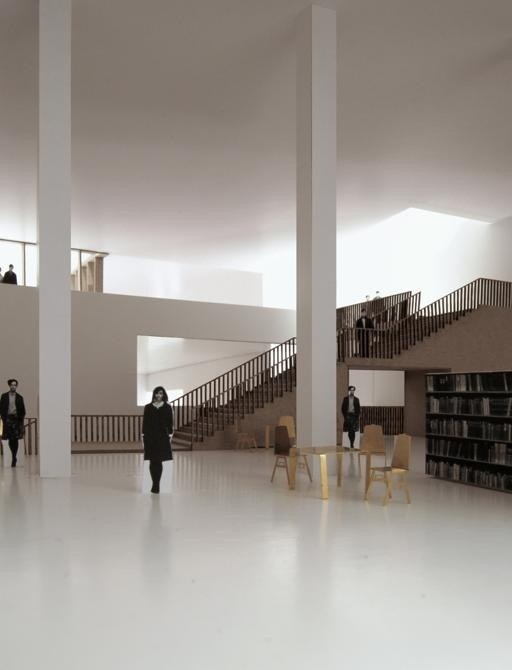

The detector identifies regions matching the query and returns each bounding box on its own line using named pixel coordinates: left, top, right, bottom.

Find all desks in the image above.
left=288, top=446, right=372, bottom=500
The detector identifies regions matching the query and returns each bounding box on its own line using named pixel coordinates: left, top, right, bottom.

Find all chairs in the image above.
left=278, top=416, right=296, bottom=439
left=358, top=424, right=387, bottom=478
left=271, top=426, right=312, bottom=487
left=235, top=415, right=258, bottom=450
left=365, top=433, right=412, bottom=506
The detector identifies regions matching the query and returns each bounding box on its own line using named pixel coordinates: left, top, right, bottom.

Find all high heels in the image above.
left=11, top=459, right=17, bottom=466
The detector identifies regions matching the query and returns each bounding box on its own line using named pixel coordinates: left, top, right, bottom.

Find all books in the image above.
left=426, top=372, right=512, bottom=490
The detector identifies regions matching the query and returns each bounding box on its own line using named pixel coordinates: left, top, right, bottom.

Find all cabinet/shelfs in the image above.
left=424, top=370, right=511, bottom=494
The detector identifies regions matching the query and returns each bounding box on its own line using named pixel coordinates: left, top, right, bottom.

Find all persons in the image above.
left=374, top=291, right=381, bottom=301
left=355, top=308, right=374, bottom=357
left=366, top=295, right=370, bottom=301
left=3, top=264, right=16, bottom=284
left=0, top=379, right=25, bottom=467
left=342, top=386, right=360, bottom=448
left=142, top=387, right=173, bottom=493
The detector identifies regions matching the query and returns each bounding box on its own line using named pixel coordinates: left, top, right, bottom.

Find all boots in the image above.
left=151, top=474, right=161, bottom=493
